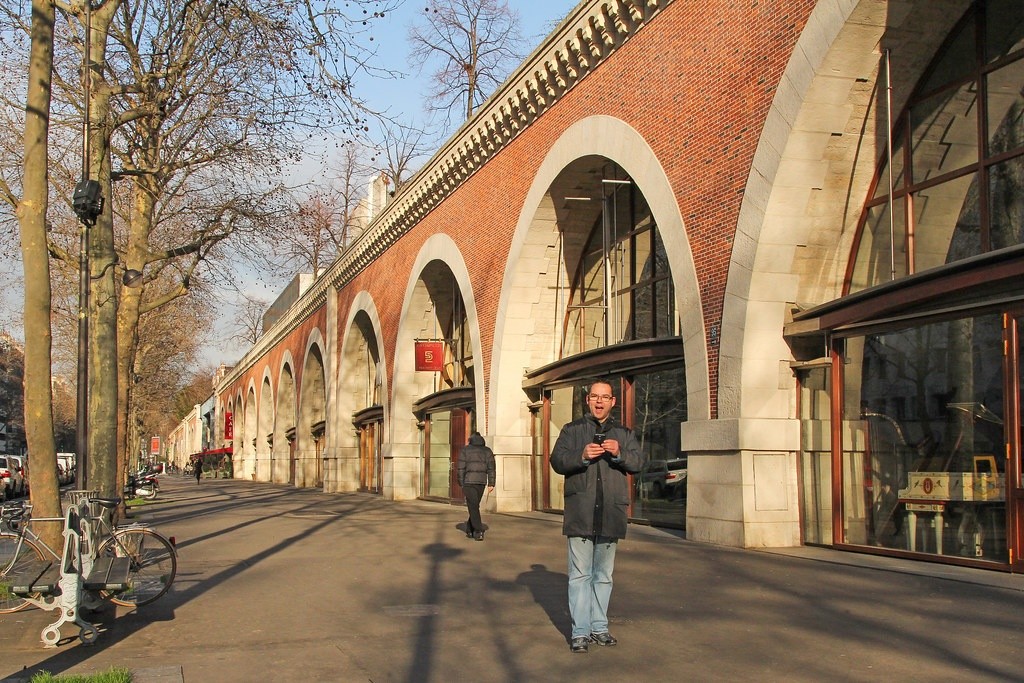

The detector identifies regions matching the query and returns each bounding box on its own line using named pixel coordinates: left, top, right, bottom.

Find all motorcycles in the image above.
left=124, top=464, right=163, bottom=500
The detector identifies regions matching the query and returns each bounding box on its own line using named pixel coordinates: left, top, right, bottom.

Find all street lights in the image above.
left=73, top=1, right=145, bottom=518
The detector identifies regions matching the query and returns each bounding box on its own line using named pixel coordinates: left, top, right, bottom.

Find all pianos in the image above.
left=897, top=471, right=1024, bottom=555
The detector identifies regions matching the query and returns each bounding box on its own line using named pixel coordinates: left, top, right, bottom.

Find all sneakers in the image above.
left=571, top=636, right=589, bottom=653
left=589, top=630, right=617, bottom=645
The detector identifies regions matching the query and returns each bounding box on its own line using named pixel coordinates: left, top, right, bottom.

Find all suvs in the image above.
left=634, top=457, right=689, bottom=496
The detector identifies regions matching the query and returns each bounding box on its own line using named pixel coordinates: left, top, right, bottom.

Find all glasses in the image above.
left=588, top=394, right=613, bottom=402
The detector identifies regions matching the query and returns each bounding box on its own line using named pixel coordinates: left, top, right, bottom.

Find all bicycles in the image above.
left=0, top=498, right=180, bottom=614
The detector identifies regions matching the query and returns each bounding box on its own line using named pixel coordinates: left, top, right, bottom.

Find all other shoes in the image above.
left=466, top=532, right=474, bottom=538
left=476, top=533, right=483, bottom=541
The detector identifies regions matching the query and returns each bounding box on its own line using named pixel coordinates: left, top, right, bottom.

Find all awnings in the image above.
left=199, top=446, right=234, bottom=457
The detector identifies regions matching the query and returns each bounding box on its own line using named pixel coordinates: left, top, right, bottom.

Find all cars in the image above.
left=56, top=453, right=77, bottom=485
left=0, top=451, right=30, bottom=501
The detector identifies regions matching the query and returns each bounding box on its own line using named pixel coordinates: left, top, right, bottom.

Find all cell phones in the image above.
left=593, top=434, right=606, bottom=447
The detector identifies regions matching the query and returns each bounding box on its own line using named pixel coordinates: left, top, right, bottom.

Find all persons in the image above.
left=548, top=377, right=644, bottom=654
left=457, top=432, right=496, bottom=542
left=195, top=459, right=202, bottom=485
